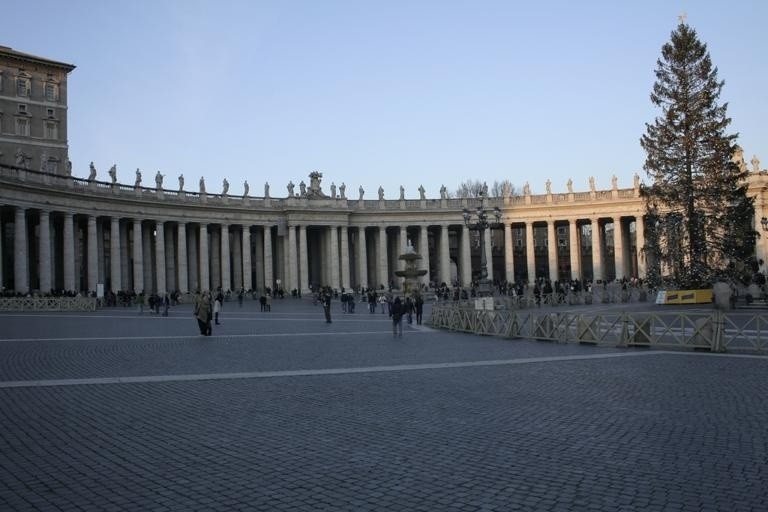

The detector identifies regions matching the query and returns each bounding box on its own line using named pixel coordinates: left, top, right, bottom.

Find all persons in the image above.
left=329, top=168, right=664, bottom=200
left=0, top=287, right=181, bottom=318
left=429, top=272, right=657, bottom=306
left=14, top=150, right=307, bottom=199
left=309, top=280, right=427, bottom=336
left=227, top=284, right=300, bottom=312
left=192, top=285, right=225, bottom=336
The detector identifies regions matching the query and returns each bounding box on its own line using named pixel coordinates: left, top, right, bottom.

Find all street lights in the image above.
left=460, top=183, right=504, bottom=322
left=760, top=215, right=768, bottom=240
